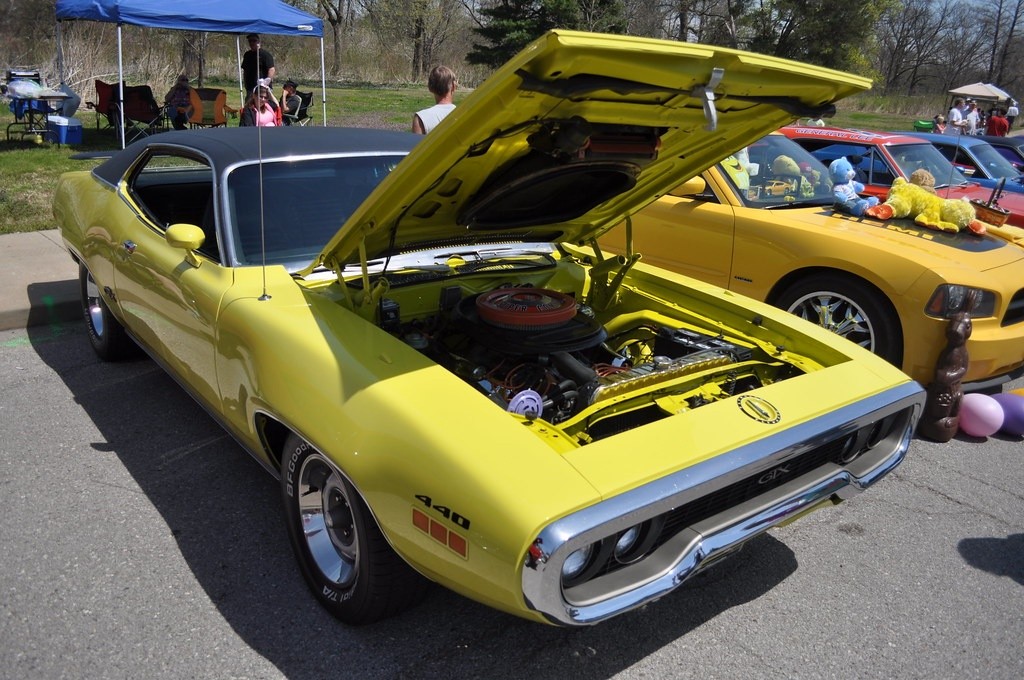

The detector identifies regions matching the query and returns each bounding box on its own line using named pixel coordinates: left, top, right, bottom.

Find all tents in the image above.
left=55, top=0, right=327, bottom=151
left=943, top=81, right=1018, bottom=116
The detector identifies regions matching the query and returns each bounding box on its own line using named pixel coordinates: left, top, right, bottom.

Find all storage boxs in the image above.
left=44, top=115, right=83, bottom=145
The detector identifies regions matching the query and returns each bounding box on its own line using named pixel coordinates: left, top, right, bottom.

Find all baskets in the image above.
left=968, top=177, right=1012, bottom=228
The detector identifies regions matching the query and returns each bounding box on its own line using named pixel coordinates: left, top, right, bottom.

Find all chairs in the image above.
left=278, top=190, right=348, bottom=248
left=847, top=153, right=868, bottom=184
left=5, top=71, right=314, bottom=144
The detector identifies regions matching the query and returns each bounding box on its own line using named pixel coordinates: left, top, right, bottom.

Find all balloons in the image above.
left=958, top=393, right=1004, bottom=437
left=989, top=393, right=1024, bottom=437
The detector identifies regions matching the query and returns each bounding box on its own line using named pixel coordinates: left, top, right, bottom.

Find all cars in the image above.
left=774, top=125, right=1024, bottom=230
left=575, top=129, right=1024, bottom=404
left=807, top=130, right=1024, bottom=196
left=960, top=133, right=1024, bottom=176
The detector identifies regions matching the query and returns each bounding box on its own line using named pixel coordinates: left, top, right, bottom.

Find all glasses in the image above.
left=260, top=95, right=268, bottom=99
left=180, top=78, right=188, bottom=82
left=248, top=39, right=259, bottom=43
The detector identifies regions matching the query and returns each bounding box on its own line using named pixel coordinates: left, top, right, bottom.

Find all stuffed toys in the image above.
left=721, top=156, right=750, bottom=198
left=772, top=155, right=820, bottom=198
left=869, top=169, right=986, bottom=237
left=828, top=156, right=879, bottom=216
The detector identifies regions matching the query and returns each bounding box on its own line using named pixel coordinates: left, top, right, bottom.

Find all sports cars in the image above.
left=49, top=26, right=930, bottom=631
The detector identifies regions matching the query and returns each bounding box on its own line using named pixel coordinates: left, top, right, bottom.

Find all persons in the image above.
left=280, top=81, right=299, bottom=128
left=240, top=32, right=275, bottom=102
left=807, top=116, right=825, bottom=127
left=164, top=75, right=200, bottom=130
left=412, top=65, right=457, bottom=135
left=935, top=97, right=1019, bottom=136
left=240, top=79, right=279, bottom=127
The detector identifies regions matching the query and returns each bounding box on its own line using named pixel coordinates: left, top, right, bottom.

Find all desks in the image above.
left=7, top=80, right=73, bottom=148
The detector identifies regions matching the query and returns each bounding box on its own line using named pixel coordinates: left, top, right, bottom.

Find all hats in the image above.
left=287, top=78, right=298, bottom=89
left=246, top=33, right=259, bottom=39
left=968, top=99, right=977, bottom=105
